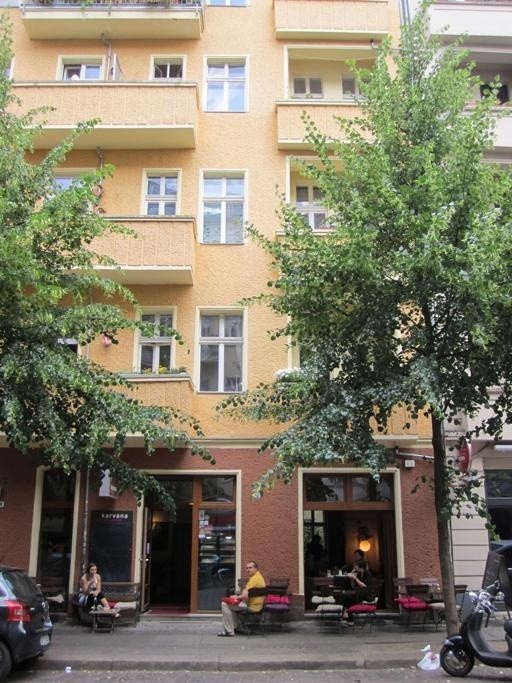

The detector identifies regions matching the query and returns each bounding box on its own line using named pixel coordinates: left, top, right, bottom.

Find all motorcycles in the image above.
left=440, top=579, right=512, bottom=677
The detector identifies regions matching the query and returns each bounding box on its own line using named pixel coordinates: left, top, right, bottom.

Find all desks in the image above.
left=89, top=609, right=120, bottom=634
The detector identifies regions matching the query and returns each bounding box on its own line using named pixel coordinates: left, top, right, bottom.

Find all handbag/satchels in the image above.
left=71, top=591, right=89, bottom=606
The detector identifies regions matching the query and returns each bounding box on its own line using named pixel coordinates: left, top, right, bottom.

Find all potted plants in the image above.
left=179, top=367, right=187, bottom=374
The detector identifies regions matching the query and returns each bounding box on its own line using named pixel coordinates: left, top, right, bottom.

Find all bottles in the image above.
left=327, top=569, right=348, bottom=577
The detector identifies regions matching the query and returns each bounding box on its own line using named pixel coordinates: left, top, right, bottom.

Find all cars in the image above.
left=0, top=563, right=55, bottom=682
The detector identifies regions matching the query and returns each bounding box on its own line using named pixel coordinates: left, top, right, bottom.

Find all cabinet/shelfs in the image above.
left=195, top=525, right=236, bottom=568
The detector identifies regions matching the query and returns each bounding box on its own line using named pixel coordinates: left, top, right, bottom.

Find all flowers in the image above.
left=273, top=367, right=315, bottom=382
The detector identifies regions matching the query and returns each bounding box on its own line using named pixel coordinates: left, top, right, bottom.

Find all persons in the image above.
left=216, top=558, right=267, bottom=637
left=343, top=547, right=370, bottom=604
left=339, top=559, right=380, bottom=627
left=305, top=533, right=328, bottom=591
left=76, top=560, right=122, bottom=624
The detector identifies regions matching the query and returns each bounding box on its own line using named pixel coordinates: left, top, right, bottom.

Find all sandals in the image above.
left=217, top=630, right=234, bottom=635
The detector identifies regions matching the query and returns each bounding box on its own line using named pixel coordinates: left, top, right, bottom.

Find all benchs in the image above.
left=72, top=581, right=141, bottom=628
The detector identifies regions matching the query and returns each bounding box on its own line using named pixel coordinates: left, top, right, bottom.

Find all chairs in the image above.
left=26, top=576, right=65, bottom=621
left=237, top=574, right=468, bottom=635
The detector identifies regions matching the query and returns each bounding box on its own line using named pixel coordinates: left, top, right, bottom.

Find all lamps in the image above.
left=357, top=531, right=371, bottom=552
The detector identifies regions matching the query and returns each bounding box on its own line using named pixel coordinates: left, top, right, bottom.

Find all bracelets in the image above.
left=354, top=577, right=357, bottom=581
